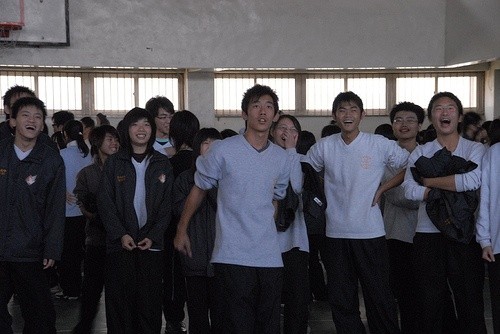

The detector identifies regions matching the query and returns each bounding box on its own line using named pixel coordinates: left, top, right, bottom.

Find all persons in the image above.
left=476, top=142, right=500, bottom=334
left=172, top=128, right=221, bottom=334
left=220, top=129, right=239, bottom=140
left=169, top=111, right=200, bottom=179
left=0, top=97, right=66, bottom=334
left=51, top=130, right=68, bottom=150
left=302, top=91, right=411, bottom=334
left=376, top=124, right=398, bottom=144
left=403, top=92, right=488, bottom=334
left=51, top=111, right=74, bottom=133
left=78, top=117, right=94, bottom=149
left=145, top=96, right=187, bottom=334
left=180, top=84, right=284, bottom=334
left=73, top=125, right=122, bottom=334
left=296, top=129, right=316, bottom=154
left=268, top=115, right=311, bottom=334
left=416, top=111, right=497, bottom=150
left=56, top=121, right=94, bottom=302
left=381, top=103, right=426, bottom=334
left=116, top=120, right=130, bottom=148
left=0, top=86, right=48, bottom=142
left=98, top=107, right=175, bottom=334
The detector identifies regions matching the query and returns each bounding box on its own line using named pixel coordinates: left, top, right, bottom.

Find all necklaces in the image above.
left=310, top=125, right=345, bottom=309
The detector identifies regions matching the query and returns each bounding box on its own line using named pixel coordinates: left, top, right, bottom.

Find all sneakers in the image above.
left=50, top=286, right=80, bottom=301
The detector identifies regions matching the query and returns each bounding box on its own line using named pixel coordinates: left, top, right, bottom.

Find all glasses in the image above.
left=275, top=125, right=298, bottom=133
left=157, top=115, right=175, bottom=119
left=52, top=123, right=57, bottom=127
left=432, top=105, right=459, bottom=112
left=131, top=123, right=151, bottom=128
left=394, top=118, right=418, bottom=124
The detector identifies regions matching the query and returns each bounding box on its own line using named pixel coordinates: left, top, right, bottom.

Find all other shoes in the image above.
left=165, top=321, right=188, bottom=334
